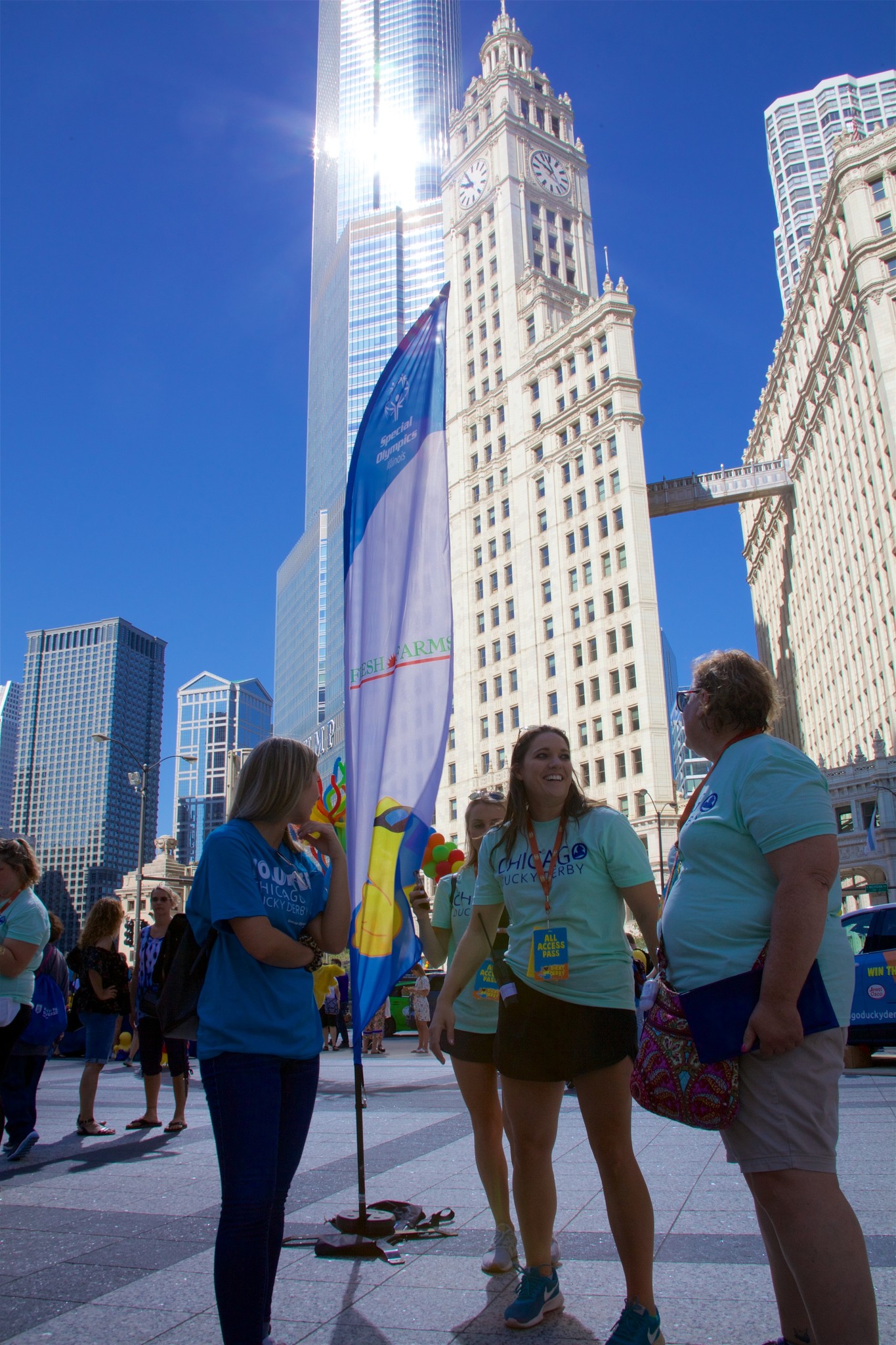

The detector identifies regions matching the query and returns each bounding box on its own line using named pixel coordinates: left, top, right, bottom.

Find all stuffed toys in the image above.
left=312, top=964, right=345, bottom=1010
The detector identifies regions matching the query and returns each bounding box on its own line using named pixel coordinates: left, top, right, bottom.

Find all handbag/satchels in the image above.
left=409, top=996, right=418, bottom=1030
left=15, top=944, right=68, bottom=1057
left=628, top=979, right=741, bottom=1131
left=676, top=959, right=840, bottom=1063
left=325, top=996, right=340, bottom=1015
left=136, top=912, right=217, bottom=1029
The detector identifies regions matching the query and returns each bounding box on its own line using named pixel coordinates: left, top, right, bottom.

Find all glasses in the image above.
left=517, top=725, right=566, bottom=742
left=676, top=688, right=702, bottom=710
left=275, top=850, right=310, bottom=889
left=151, top=897, right=172, bottom=902
left=468, top=791, right=507, bottom=807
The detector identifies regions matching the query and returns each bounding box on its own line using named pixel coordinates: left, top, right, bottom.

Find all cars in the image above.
left=383, top=969, right=446, bottom=1034
left=837, top=903, right=896, bottom=1048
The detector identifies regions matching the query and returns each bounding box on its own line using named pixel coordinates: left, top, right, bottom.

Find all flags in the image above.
left=864, top=804, right=877, bottom=856
left=341, top=305, right=453, bottom=1028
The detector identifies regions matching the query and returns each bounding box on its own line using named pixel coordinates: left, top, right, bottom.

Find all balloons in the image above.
left=291, top=757, right=346, bottom=890
left=420, top=826, right=466, bottom=883
left=424, top=961, right=429, bottom=969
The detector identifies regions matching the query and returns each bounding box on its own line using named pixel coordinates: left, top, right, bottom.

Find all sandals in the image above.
left=332, top=1046, right=339, bottom=1051
left=322, top=1046, right=329, bottom=1051
left=76, top=1114, right=107, bottom=1126
left=77, top=1118, right=116, bottom=1136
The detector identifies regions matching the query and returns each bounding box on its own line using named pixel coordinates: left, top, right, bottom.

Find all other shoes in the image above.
left=328, top=1039, right=336, bottom=1046
left=367, top=1041, right=373, bottom=1050
left=378, top=1044, right=385, bottom=1052
left=371, top=1049, right=383, bottom=1054
left=361, top=1049, right=368, bottom=1053
left=337, top=1043, right=349, bottom=1048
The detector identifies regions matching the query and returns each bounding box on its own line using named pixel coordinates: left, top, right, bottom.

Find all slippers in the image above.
left=126, top=1118, right=162, bottom=1130
left=164, top=1120, right=188, bottom=1133
left=416, top=1048, right=428, bottom=1053
left=410, top=1048, right=419, bottom=1053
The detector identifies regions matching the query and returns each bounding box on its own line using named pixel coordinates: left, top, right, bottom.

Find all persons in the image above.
left=186, top=738, right=350, bottom=1345
left=65, top=896, right=130, bottom=1136
left=3, top=911, right=69, bottom=1151
left=429, top=725, right=663, bottom=1345
left=318, top=958, right=350, bottom=1052
left=50, top=965, right=198, bottom=1062
left=126, top=886, right=192, bottom=1133
left=361, top=995, right=391, bottom=1054
left=0, top=837, right=51, bottom=1161
left=409, top=786, right=560, bottom=1275
left=657, top=646, right=880, bottom=1345
left=624, top=932, right=657, bottom=1049
left=402, top=963, right=430, bottom=1054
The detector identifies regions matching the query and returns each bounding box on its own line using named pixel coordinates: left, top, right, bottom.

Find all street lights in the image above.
left=639, top=788, right=679, bottom=898
left=91, top=733, right=198, bottom=1020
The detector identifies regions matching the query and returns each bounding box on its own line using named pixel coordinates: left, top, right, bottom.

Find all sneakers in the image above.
left=3, top=1141, right=14, bottom=1151
left=123, top=1057, right=133, bottom=1066
left=603, top=1298, right=666, bottom=1345
left=480, top=1223, right=518, bottom=1272
left=550, top=1233, right=561, bottom=1264
left=6, top=1128, right=40, bottom=1161
left=503, top=1263, right=564, bottom=1329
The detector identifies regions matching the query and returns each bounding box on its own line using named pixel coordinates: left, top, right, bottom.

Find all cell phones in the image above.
left=413, top=869, right=430, bottom=910
